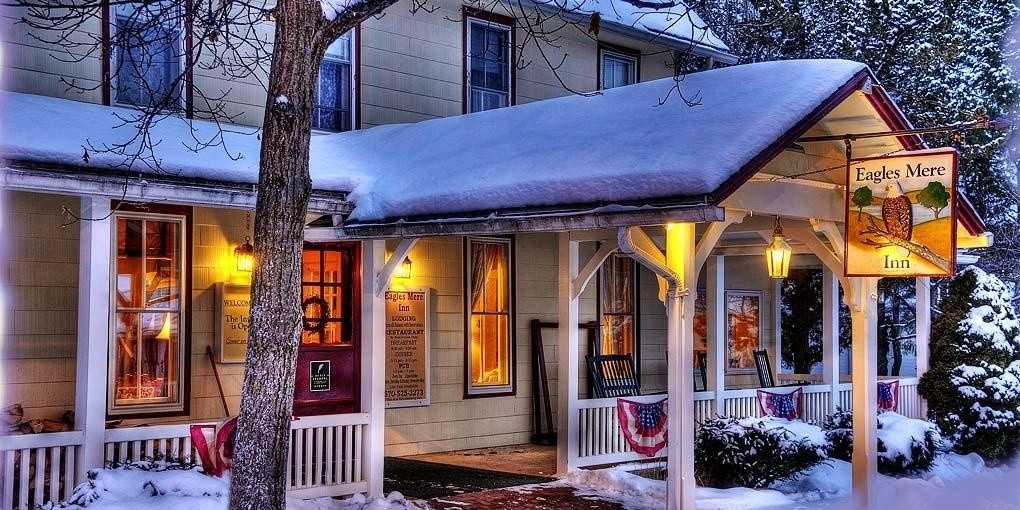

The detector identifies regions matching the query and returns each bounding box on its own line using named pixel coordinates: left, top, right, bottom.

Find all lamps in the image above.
left=394, top=237, right=411, bottom=280
left=764, top=216, right=792, bottom=279
left=234, top=211, right=255, bottom=272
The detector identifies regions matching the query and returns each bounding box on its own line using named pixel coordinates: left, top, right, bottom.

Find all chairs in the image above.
left=665, top=351, right=704, bottom=391
left=752, top=348, right=811, bottom=386
left=585, top=353, right=667, bottom=397
left=698, top=351, right=741, bottom=390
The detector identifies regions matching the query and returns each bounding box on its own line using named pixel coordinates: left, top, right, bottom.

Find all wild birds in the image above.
left=880, top=179, right=914, bottom=243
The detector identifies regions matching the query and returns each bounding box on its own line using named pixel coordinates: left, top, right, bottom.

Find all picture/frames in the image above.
left=214, top=280, right=251, bottom=364
left=693, top=288, right=708, bottom=374
left=723, top=288, right=764, bottom=376
left=386, top=284, right=431, bottom=409
